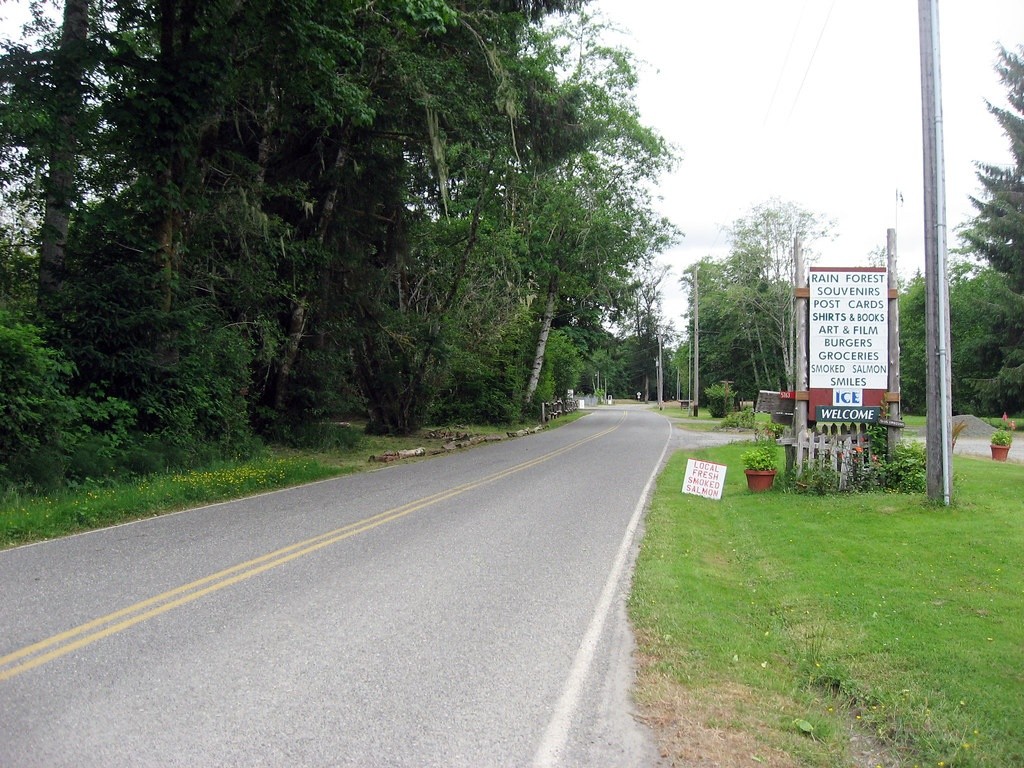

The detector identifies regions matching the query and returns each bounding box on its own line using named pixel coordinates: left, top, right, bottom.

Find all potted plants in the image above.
left=742, top=446, right=777, bottom=490
left=990, top=428, right=1012, bottom=462
left=952, top=421, right=968, bottom=452
